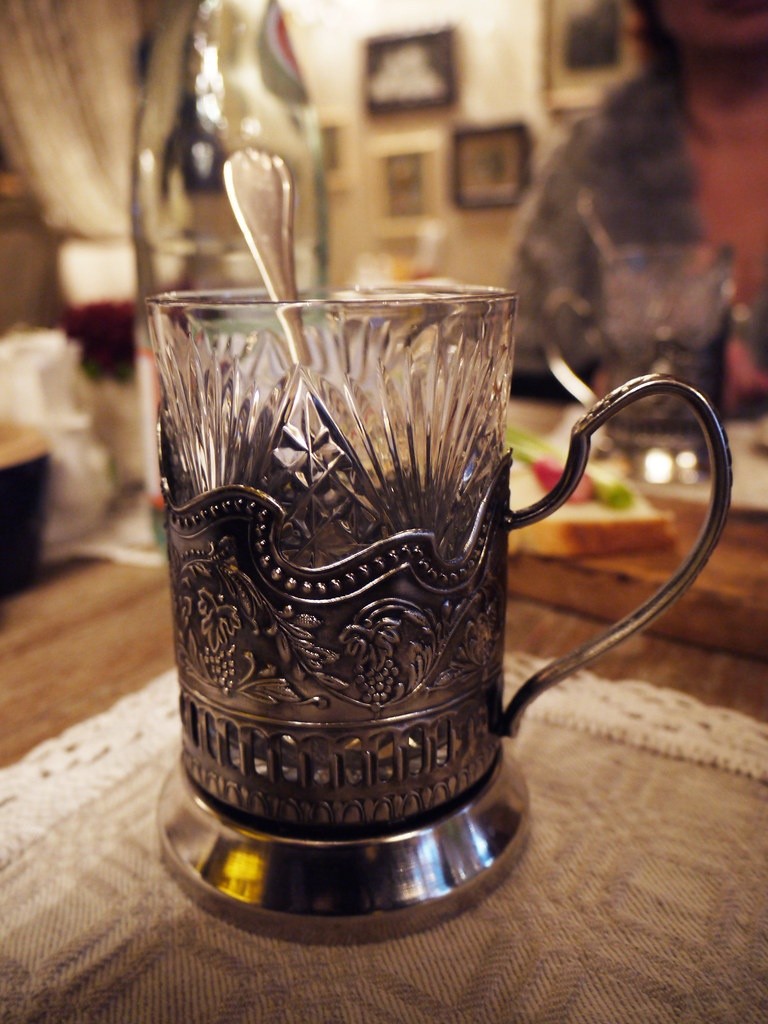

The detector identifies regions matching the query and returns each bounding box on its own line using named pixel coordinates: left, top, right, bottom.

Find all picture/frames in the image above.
left=374, top=151, right=434, bottom=215
left=454, top=124, right=532, bottom=211
left=540, top=0, right=648, bottom=118
left=364, top=29, right=456, bottom=117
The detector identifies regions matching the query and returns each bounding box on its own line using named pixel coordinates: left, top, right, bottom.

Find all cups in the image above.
left=536, top=238, right=738, bottom=488
left=142, top=284, right=734, bottom=943
